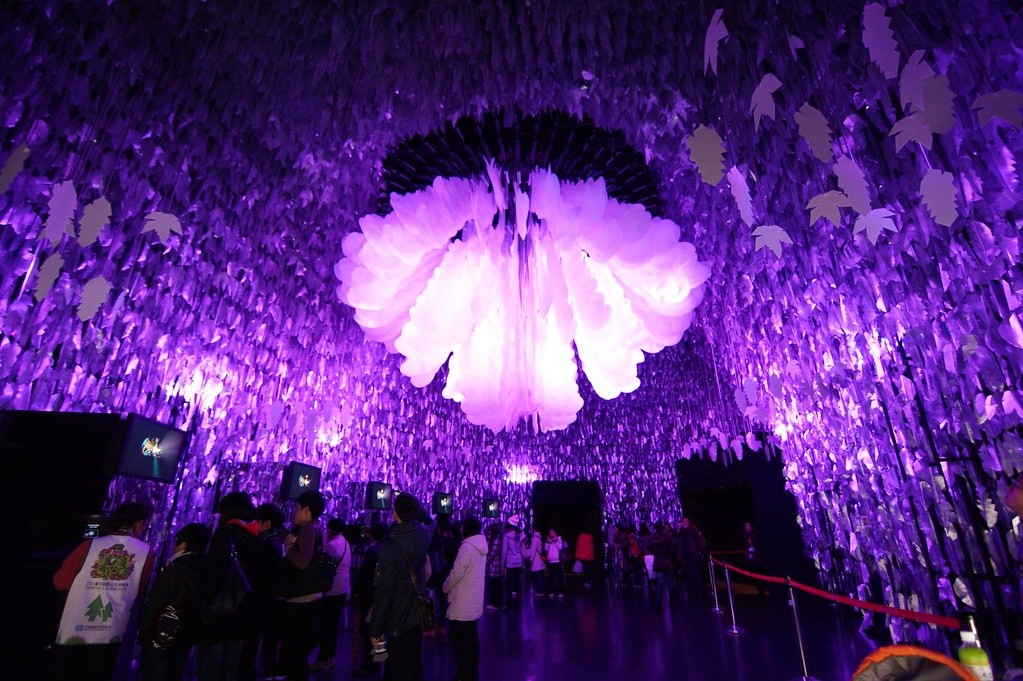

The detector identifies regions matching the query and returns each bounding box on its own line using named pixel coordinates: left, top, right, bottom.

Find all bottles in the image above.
left=959, top=631, right=993, bottom=681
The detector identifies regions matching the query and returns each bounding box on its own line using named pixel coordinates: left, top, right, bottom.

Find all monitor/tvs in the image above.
left=285, top=461, right=322, bottom=501
left=116, top=412, right=187, bottom=484
left=483, top=499, right=500, bottom=518
left=364, top=481, right=393, bottom=510
left=432, top=493, right=454, bottom=514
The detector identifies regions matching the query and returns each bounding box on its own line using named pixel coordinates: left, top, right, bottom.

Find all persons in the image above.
left=51, top=491, right=708, bottom=681
left=738, top=521, right=769, bottom=597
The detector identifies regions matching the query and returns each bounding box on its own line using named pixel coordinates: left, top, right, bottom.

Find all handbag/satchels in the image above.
left=419, top=586, right=440, bottom=631
left=282, top=520, right=336, bottom=599
left=156, top=596, right=195, bottom=647
left=207, top=530, right=251, bottom=619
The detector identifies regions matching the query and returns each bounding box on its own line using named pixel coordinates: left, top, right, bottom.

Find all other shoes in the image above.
left=352, top=667, right=369, bottom=677
left=308, top=659, right=331, bottom=671
left=487, top=605, right=499, bottom=610
left=329, top=657, right=337, bottom=666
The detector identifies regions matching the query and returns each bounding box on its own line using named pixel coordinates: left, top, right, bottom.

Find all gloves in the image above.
left=371, top=639, right=389, bottom=662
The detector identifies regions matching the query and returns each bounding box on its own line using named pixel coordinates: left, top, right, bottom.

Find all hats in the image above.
left=328, top=518, right=345, bottom=533
left=507, top=513, right=520, bottom=527
left=395, top=493, right=420, bottom=522
